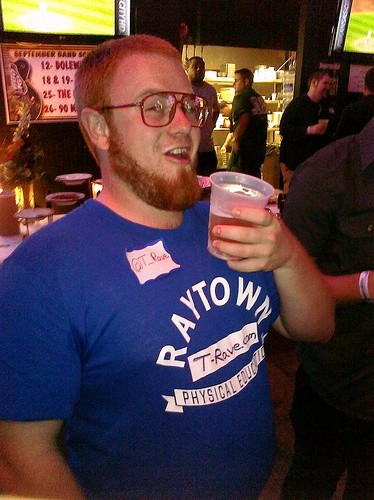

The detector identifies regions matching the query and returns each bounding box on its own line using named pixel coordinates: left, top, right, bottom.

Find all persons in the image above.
left=0, top=36, right=337, bottom=500
left=184, top=57, right=374, bottom=221
left=282, top=117, right=374, bottom=500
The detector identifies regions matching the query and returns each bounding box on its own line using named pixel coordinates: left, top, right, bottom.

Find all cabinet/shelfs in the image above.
left=203, top=53, right=296, bottom=189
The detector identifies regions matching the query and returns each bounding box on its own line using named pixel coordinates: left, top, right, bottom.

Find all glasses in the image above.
left=94, top=91, right=210, bottom=128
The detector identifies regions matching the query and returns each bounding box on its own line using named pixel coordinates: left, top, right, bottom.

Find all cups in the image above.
left=206, top=171, right=274, bottom=262
left=318, top=119, right=329, bottom=133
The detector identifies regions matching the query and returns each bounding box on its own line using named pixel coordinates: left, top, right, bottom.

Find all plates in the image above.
left=55, top=173, right=93, bottom=186
left=14, top=208, right=55, bottom=221
left=45, top=192, right=85, bottom=205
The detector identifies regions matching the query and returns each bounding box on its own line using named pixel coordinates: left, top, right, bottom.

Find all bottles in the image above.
left=267, top=111, right=272, bottom=128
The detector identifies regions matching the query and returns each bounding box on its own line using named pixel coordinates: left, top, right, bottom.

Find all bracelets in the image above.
left=307, top=125, right=312, bottom=135
left=359, top=271, right=371, bottom=303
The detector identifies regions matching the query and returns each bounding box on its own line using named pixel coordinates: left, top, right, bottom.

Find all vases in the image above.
left=13, top=179, right=47, bottom=210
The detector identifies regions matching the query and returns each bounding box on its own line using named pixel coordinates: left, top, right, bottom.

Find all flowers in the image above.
left=0, top=96, right=46, bottom=191
